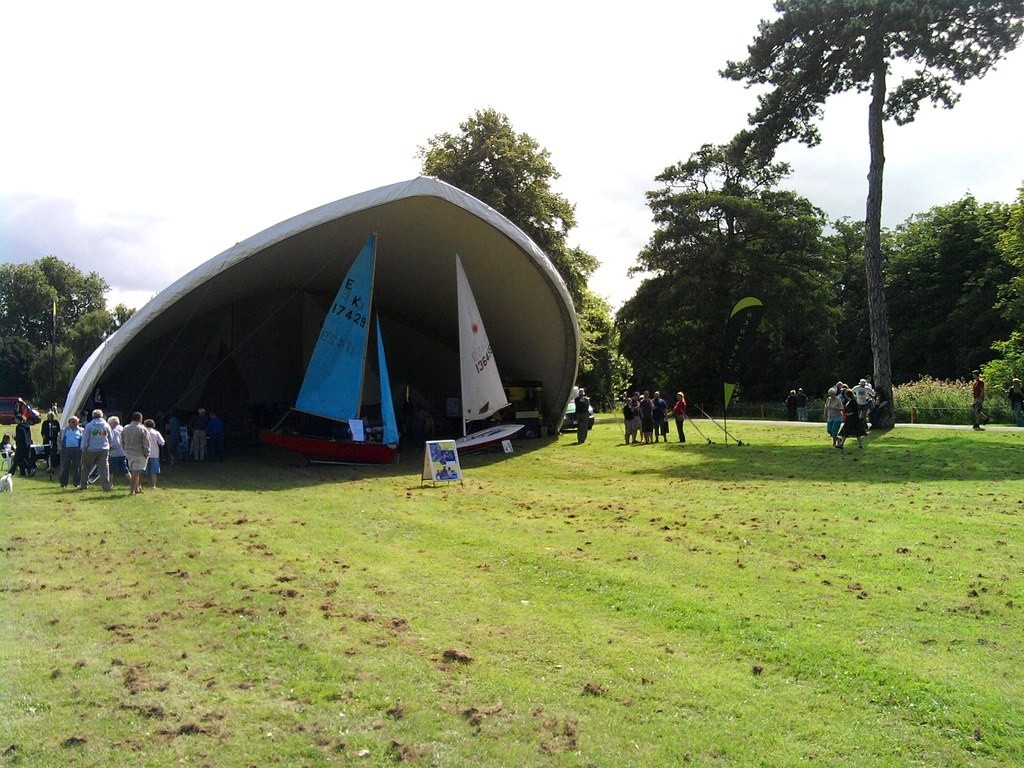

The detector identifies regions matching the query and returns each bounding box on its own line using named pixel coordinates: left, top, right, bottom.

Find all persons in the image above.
left=1006, top=379, right=1024, bottom=427
left=667, top=392, right=686, bottom=443
left=205, top=411, right=224, bottom=462
left=145, top=419, right=165, bottom=490
left=191, top=408, right=207, bottom=461
left=823, top=375, right=888, bottom=449
left=0, top=414, right=38, bottom=478
left=575, top=389, right=589, bottom=444
left=158, top=412, right=180, bottom=464
left=786, top=388, right=808, bottom=422
left=403, top=396, right=434, bottom=449
left=119, top=412, right=150, bottom=496
left=624, top=391, right=667, bottom=445
left=972, top=370, right=984, bottom=429
left=77, top=409, right=113, bottom=492
left=77, top=411, right=100, bottom=485
left=41, top=412, right=60, bottom=474
left=53, top=403, right=58, bottom=414
left=107, top=416, right=132, bottom=489
left=60, top=416, right=85, bottom=487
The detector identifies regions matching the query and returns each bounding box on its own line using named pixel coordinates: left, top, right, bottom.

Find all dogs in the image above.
left=0, top=474, right=13, bottom=492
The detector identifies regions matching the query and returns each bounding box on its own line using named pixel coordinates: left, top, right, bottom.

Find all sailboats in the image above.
left=451, top=251, right=528, bottom=457
left=255, top=229, right=403, bottom=464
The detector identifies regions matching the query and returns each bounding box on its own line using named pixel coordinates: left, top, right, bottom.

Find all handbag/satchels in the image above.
left=677, top=415, right=685, bottom=420
left=660, top=420, right=669, bottom=435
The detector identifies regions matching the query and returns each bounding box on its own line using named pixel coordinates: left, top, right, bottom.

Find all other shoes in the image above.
left=983, top=418, right=990, bottom=425
left=136, top=489, right=144, bottom=494
left=973, top=424, right=980, bottom=428
left=835, top=445, right=843, bottom=450
left=128, top=493, right=138, bottom=498
left=859, top=445, right=864, bottom=449
left=650, top=441, right=653, bottom=444
left=76, top=486, right=81, bottom=493
left=645, top=441, right=650, bottom=444
left=60, top=486, right=67, bottom=492
left=30, top=468, right=36, bottom=477
left=110, top=485, right=114, bottom=490
left=16, top=474, right=26, bottom=478
left=641, top=441, right=644, bottom=444
left=149, top=485, right=156, bottom=491
left=632, top=439, right=640, bottom=444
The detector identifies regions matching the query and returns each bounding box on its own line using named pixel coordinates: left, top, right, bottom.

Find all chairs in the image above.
left=0, top=452, right=13, bottom=471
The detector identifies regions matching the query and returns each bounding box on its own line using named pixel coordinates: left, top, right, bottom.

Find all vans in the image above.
left=0, top=396, right=42, bottom=425
left=560, top=385, right=596, bottom=431
